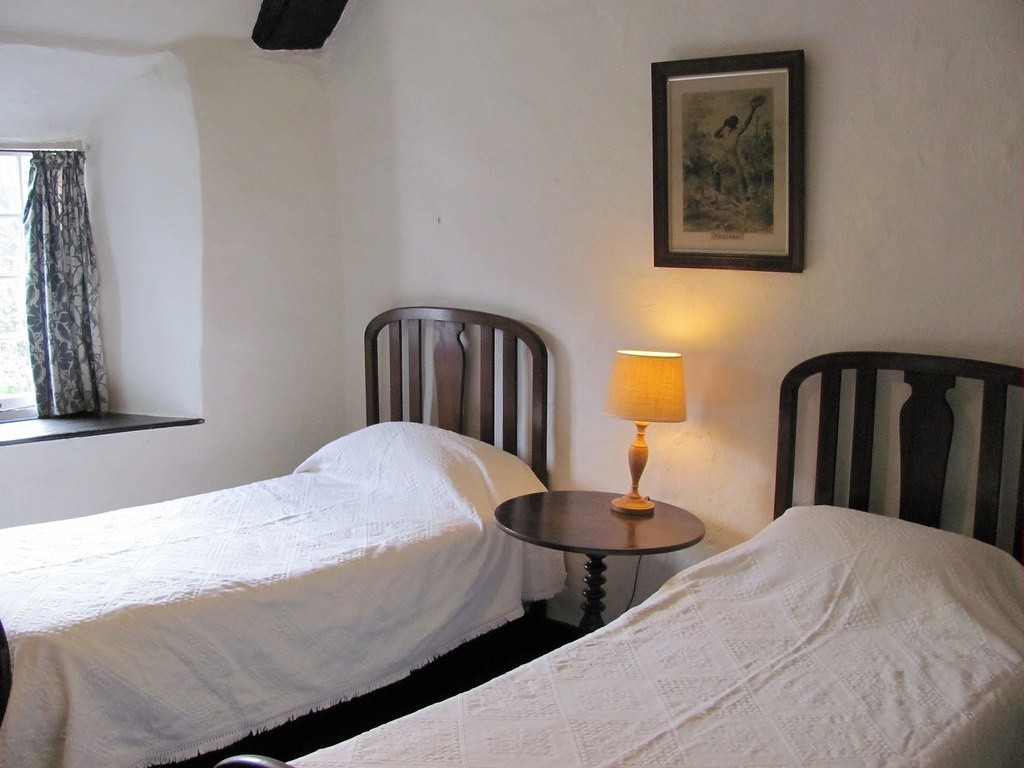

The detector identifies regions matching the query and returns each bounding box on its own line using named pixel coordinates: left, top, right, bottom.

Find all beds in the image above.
left=288, top=505, right=1024, bottom=768
left=1, top=420, right=536, bottom=766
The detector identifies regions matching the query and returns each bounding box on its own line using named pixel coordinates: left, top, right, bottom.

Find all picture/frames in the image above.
left=650, top=47, right=806, bottom=274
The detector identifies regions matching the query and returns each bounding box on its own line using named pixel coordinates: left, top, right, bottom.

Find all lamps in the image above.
left=604, top=350, right=687, bottom=517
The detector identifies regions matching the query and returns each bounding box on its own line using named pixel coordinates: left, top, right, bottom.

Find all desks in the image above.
left=495, top=490, right=706, bottom=636
